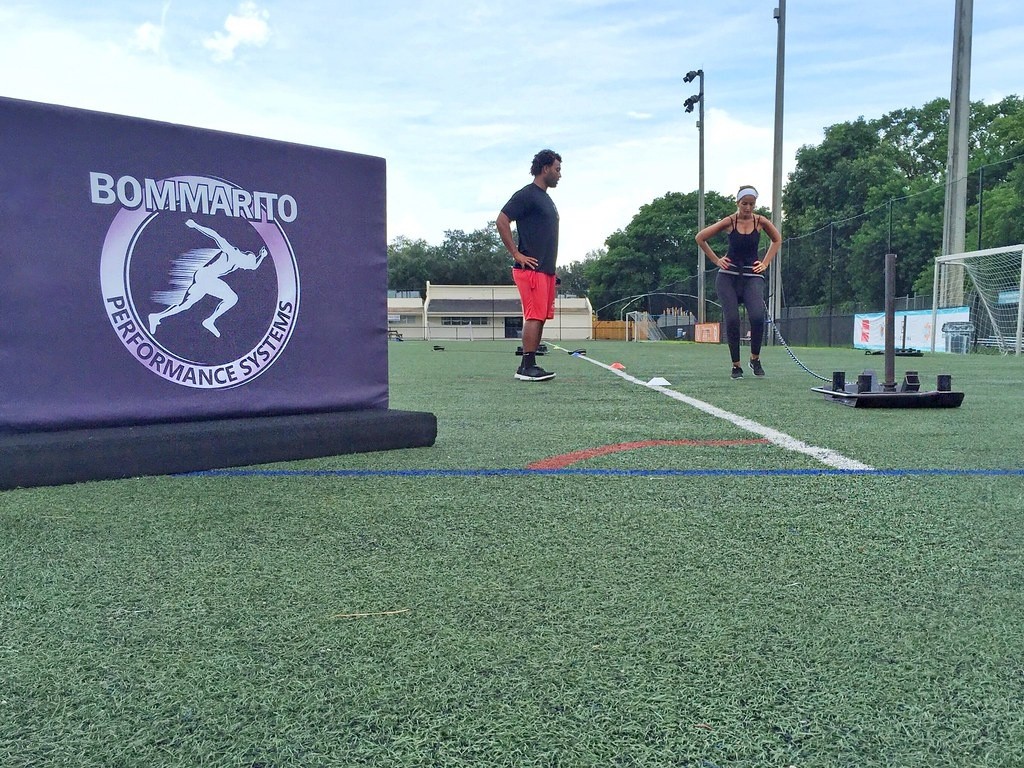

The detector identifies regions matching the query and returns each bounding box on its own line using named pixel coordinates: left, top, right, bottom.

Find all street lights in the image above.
left=682, top=68, right=704, bottom=323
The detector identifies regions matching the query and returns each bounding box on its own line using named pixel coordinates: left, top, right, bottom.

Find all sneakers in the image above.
left=519, top=364, right=556, bottom=381
left=514, top=366, right=523, bottom=378
left=748, top=357, right=766, bottom=377
left=730, top=366, right=744, bottom=380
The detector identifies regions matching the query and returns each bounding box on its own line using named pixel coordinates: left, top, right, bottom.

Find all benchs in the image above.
left=388, top=333, right=403, bottom=339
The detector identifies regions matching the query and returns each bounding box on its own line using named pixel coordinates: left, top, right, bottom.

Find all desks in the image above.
left=387, top=331, right=398, bottom=337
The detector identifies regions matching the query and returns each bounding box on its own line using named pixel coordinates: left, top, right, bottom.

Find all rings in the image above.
left=760, top=267, right=762, bottom=270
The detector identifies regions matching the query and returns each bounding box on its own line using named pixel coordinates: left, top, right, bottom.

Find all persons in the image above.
left=696, top=185, right=781, bottom=378
left=496, top=149, right=563, bottom=380
